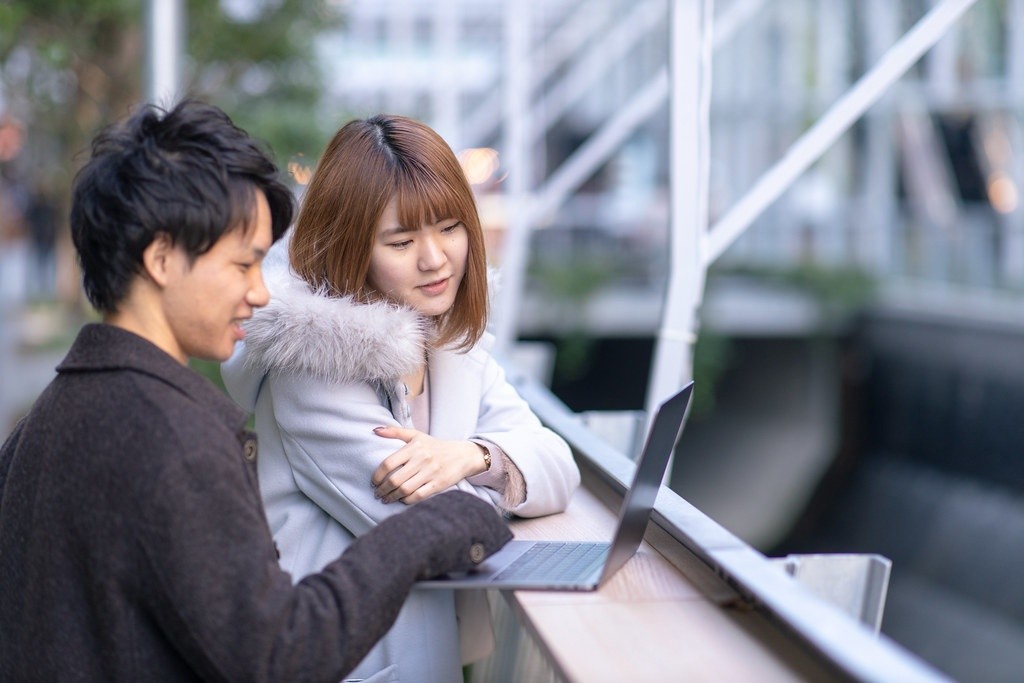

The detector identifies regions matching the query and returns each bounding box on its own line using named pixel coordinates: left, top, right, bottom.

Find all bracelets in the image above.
left=484, top=448, right=491, bottom=470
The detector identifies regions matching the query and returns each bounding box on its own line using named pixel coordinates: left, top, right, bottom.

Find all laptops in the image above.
left=410, top=379, right=696, bottom=592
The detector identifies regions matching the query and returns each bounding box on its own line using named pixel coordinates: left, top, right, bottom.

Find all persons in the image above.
left=1, top=93, right=514, bottom=683
left=219, top=115, right=580, bottom=683
